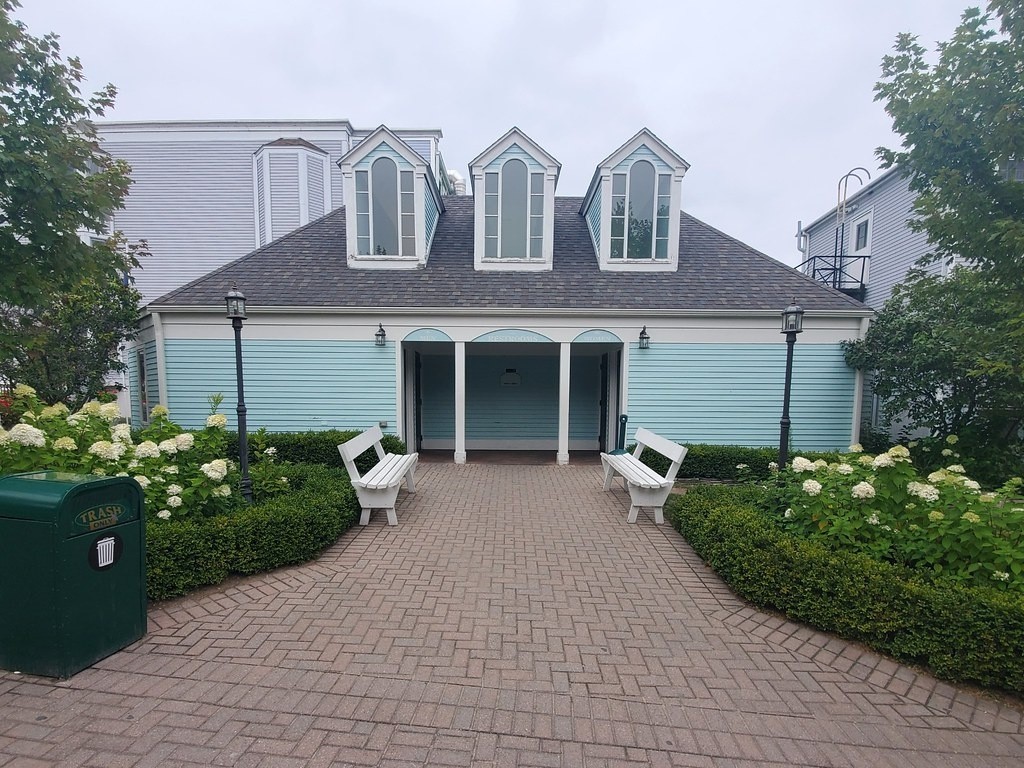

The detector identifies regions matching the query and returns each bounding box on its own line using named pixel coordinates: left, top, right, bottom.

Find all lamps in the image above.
left=639, top=325, right=651, bottom=349
left=375, top=322, right=386, bottom=345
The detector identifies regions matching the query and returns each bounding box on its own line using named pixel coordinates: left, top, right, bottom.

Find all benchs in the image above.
left=337, top=425, right=419, bottom=526
left=600, top=428, right=688, bottom=525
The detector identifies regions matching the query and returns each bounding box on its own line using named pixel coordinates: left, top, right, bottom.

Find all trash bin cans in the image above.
left=0, top=471, right=148, bottom=677
